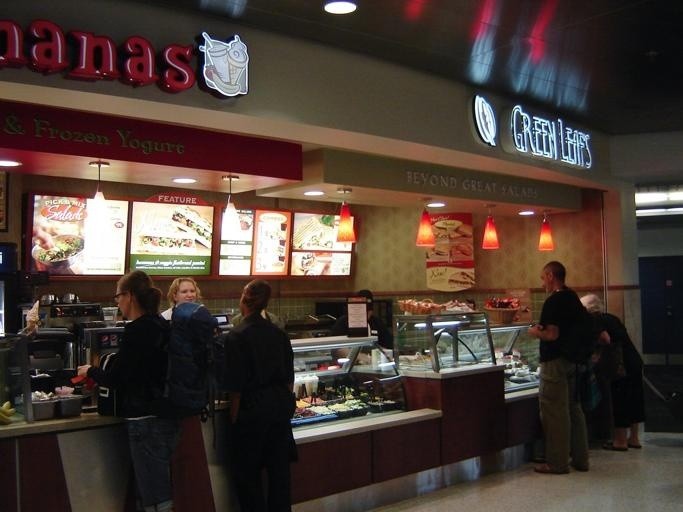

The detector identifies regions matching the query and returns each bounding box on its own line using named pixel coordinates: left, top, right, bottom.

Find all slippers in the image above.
left=603, top=438, right=628, bottom=451
left=625, top=441, right=641, bottom=448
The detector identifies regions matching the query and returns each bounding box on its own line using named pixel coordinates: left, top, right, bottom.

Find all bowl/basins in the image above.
left=32, top=233, right=86, bottom=269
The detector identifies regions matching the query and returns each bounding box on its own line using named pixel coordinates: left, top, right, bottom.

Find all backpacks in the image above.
left=127, top=320, right=211, bottom=419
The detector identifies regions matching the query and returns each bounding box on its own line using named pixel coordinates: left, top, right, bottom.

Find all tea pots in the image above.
left=41, top=292, right=62, bottom=304
left=63, top=292, right=80, bottom=304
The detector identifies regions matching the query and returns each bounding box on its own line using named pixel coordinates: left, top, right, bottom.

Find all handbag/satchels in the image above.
left=98, top=353, right=126, bottom=417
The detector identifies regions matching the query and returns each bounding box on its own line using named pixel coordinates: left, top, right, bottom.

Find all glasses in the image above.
left=112, top=291, right=127, bottom=303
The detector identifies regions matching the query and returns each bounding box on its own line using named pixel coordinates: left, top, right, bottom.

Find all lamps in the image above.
left=336, top=188, right=356, bottom=243
left=220, top=175, right=244, bottom=241
left=482, top=203, right=499, bottom=250
left=85, top=161, right=112, bottom=228
left=415, top=196, right=437, bottom=247
left=538, top=210, right=554, bottom=250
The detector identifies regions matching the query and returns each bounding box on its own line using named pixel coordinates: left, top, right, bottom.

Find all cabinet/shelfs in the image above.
left=170, top=401, right=237, bottom=511
left=504, top=390, right=539, bottom=451
left=392, top=311, right=497, bottom=372
left=457, top=327, right=542, bottom=392
left=407, top=367, right=505, bottom=457
left=0, top=426, right=132, bottom=512
left=292, top=337, right=407, bottom=426
left=289, top=407, right=446, bottom=502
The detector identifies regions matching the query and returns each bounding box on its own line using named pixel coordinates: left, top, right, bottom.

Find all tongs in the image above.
left=300, top=382, right=309, bottom=397
left=310, top=390, right=316, bottom=407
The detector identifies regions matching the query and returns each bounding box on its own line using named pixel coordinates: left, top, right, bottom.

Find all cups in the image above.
left=101, top=306, right=120, bottom=327
left=381, top=350, right=394, bottom=372
left=372, top=349, right=381, bottom=370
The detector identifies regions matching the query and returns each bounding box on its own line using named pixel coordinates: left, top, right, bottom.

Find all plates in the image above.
left=432, top=220, right=465, bottom=230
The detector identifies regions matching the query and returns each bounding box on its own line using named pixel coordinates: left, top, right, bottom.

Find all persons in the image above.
left=0, top=324, right=38, bottom=348
left=580, top=294, right=645, bottom=451
left=331, top=290, right=390, bottom=364
left=94, top=271, right=181, bottom=511
left=223, top=278, right=298, bottom=512
left=229, top=301, right=287, bottom=334
left=527, top=261, right=591, bottom=473
left=161, top=278, right=201, bottom=321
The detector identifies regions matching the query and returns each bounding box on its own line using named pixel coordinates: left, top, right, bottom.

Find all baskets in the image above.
left=398, top=299, right=446, bottom=315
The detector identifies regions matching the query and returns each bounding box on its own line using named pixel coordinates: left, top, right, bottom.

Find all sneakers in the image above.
left=534, top=462, right=553, bottom=474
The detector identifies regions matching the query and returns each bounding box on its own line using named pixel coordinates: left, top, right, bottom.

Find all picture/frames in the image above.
left=0, top=171, right=9, bottom=233
